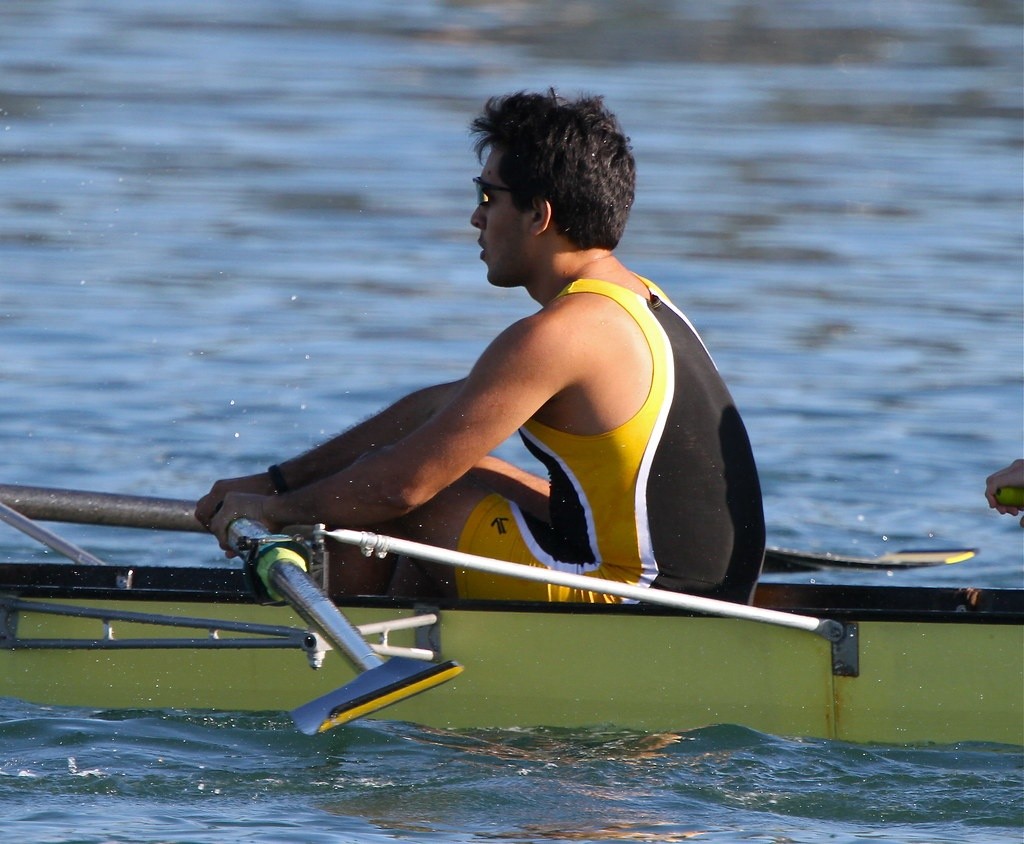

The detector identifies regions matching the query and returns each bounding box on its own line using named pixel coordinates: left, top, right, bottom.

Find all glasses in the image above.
left=472, top=176, right=547, bottom=208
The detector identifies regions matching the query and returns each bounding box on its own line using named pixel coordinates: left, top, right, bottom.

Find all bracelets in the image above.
left=267, top=463, right=290, bottom=497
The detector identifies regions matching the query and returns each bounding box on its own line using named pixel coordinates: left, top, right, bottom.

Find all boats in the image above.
left=0, top=554, right=1023, bottom=751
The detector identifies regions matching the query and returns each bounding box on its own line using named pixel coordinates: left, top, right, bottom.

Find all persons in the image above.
left=982, top=458, right=1023, bottom=517
left=193, top=85, right=767, bottom=620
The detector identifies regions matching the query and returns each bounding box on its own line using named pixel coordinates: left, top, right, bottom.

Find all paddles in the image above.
left=208, top=503, right=466, bottom=738
left=993, top=486, right=1024, bottom=508
left=763, top=545, right=981, bottom=570
